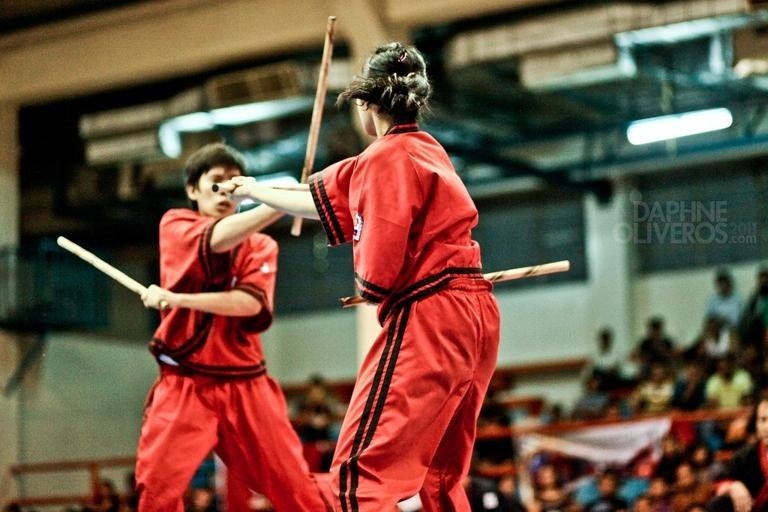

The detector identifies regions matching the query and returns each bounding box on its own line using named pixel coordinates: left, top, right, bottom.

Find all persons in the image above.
left=202, top=37, right=502, bottom=512
left=130, top=140, right=337, bottom=512
